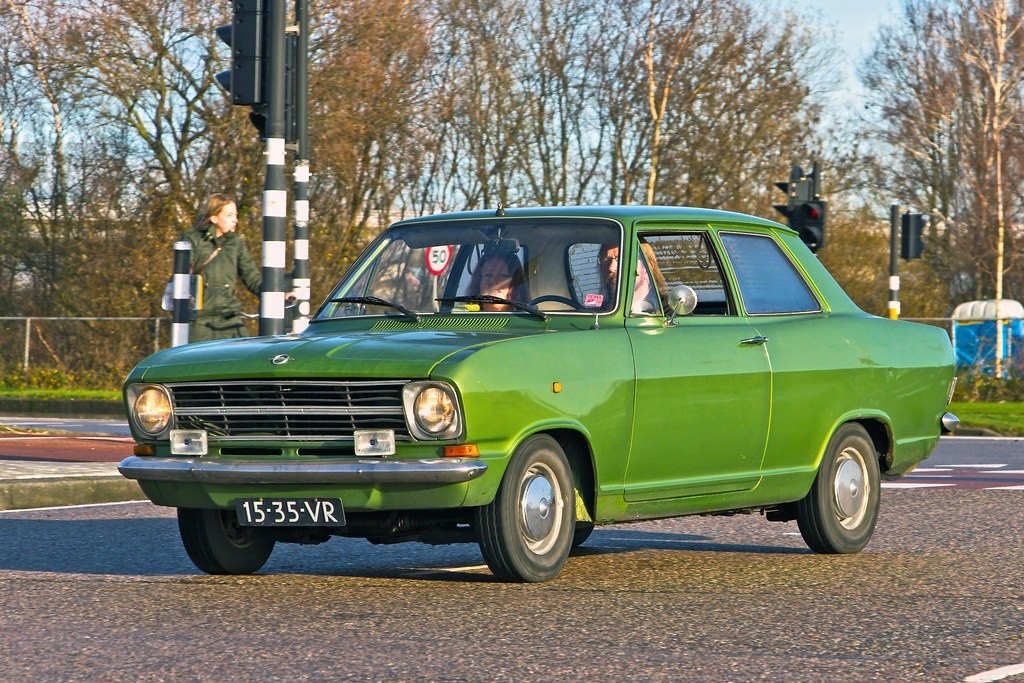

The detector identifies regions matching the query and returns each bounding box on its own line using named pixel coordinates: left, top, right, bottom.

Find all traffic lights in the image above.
left=772, top=167, right=805, bottom=231
left=215, top=0, right=259, bottom=107
left=796, top=199, right=828, bottom=251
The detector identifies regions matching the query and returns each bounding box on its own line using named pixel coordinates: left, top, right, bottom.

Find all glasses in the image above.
left=597, top=256, right=618, bottom=264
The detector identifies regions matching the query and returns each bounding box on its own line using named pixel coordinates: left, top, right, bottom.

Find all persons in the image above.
left=183, top=193, right=261, bottom=343
left=467, top=252, right=531, bottom=313
left=598, top=236, right=694, bottom=317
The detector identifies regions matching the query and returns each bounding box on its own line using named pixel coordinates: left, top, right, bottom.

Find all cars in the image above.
left=114, top=208, right=963, bottom=586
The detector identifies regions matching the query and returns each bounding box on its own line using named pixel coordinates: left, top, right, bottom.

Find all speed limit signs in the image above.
left=426, top=245, right=453, bottom=277
